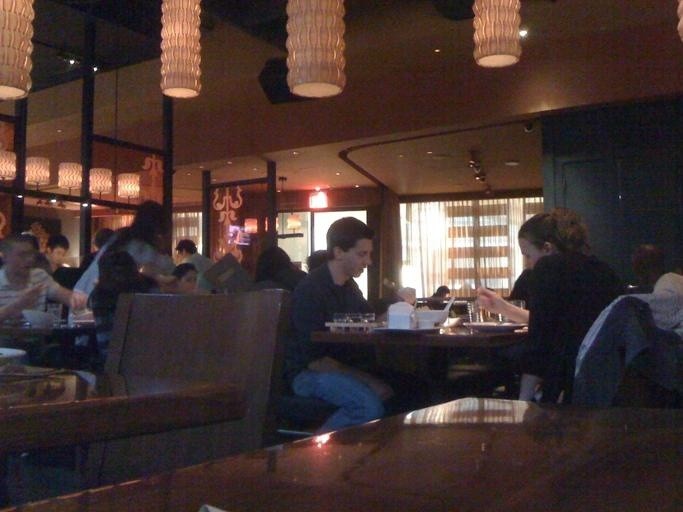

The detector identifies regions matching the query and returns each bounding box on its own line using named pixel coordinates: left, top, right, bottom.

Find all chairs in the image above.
left=102, top=287, right=290, bottom=487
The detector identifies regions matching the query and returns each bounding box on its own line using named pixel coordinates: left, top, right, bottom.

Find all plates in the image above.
left=463, top=322, right=528, bottom=331
left=374, top=326, right=440, bottom=333
left=0, top=347, right=27, bottom=360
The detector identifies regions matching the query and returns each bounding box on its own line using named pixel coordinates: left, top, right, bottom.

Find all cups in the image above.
left=509, top=300, right=526, bottom=309
left=467, top=301, right=483, bottom=322
left=333, top=313, right=376, bottom=335
left=47, top=303, right=63, bottom=327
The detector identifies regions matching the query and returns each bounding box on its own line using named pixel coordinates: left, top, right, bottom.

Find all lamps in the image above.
left=471, top=0, right=522, bottom=68
left=0, top=150, right=143, bottom=211
left=160, top=0, right=202, bottom=98
left=285, top=0, right=346, bottom=99
left=0, top=0, right=35, bottom=100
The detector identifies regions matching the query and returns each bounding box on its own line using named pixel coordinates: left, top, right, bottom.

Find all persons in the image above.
left=87, top=251, right=162, bottom=377
left=284, top=216, right=416, bottom=437
left=428, top=285, right=449, bottom=309
left=477, top=206, right=626, bottom=404
left=0, top=199, right=327, bottom=338
left=632, top=244, right=682, bottom=336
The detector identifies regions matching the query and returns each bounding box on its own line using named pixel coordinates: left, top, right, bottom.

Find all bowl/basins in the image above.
left=20, top=310, right=56, bottom=328
left=415, top=310, right=449, bottom=327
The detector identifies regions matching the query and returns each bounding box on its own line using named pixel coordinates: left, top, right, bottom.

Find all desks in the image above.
left=0, top=316, right=683, bottom=512
